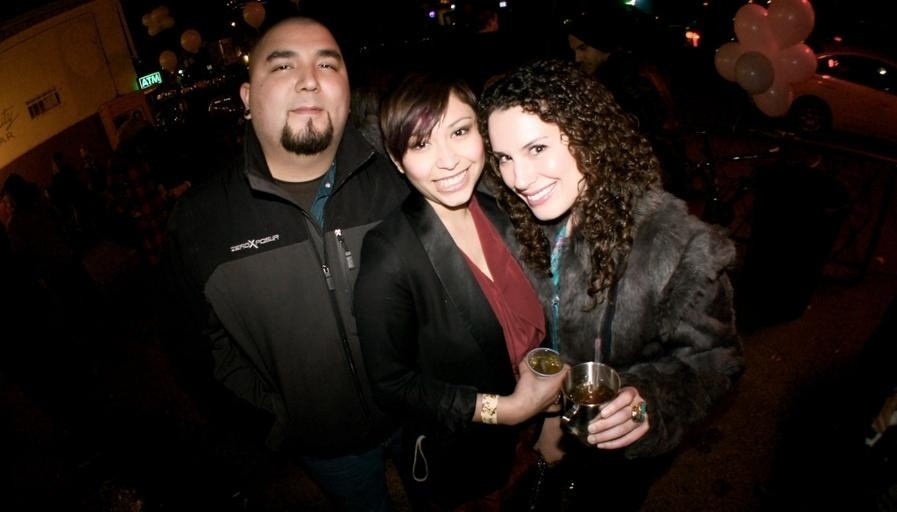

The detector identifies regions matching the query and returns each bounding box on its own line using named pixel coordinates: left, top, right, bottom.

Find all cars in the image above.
left=785, top=46, right=895, bottom=144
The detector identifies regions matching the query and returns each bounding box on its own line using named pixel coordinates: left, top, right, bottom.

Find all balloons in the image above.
left=712, top=0, right=820, bottom=117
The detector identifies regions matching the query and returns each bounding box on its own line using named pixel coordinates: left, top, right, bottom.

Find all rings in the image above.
left=632, top=398, right=646, bottom=422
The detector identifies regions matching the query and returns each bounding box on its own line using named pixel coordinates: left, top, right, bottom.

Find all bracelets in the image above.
left=477, top=390, right=500, bottom=423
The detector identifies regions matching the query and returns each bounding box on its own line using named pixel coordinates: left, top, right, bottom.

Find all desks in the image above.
left=148, top=75, right=241, bottom=135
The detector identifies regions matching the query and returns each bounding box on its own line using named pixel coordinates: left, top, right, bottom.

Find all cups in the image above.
left=559, top=361, right=622, bottom=447
left=524, top=346, right=565, bottom=378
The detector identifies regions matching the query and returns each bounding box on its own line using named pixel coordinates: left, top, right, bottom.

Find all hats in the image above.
left=564, top=10, right=625, bottom=53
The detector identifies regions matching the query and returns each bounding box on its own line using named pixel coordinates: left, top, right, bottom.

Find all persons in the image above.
left=745, top=89, right=844, bottom=338
left=351, top=68, right=557, bottom=510
left=1, top=170, right=65, bottom=274
left=156, top=15, right=432, bottom=510
left=479, top=57, right=751, bottom=511
